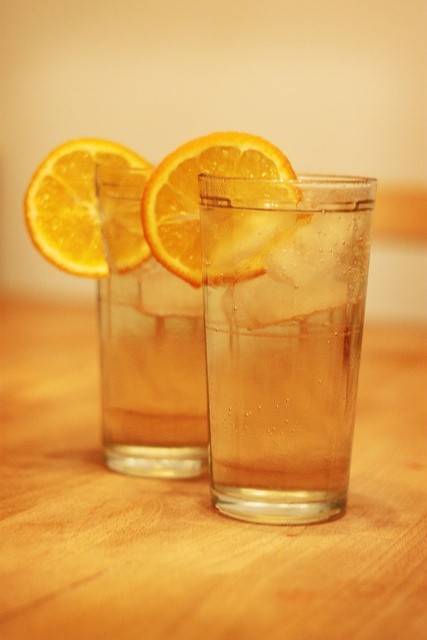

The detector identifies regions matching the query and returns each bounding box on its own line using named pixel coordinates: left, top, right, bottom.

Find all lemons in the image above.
left=23, top=135, right=156, bottom=280
left=141, top=130, right=304, bottom=290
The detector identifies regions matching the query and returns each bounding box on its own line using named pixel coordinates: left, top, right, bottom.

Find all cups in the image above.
left=94, top=163, right=210, bottom=481
left=198, top=173, right=378, bottom=525
